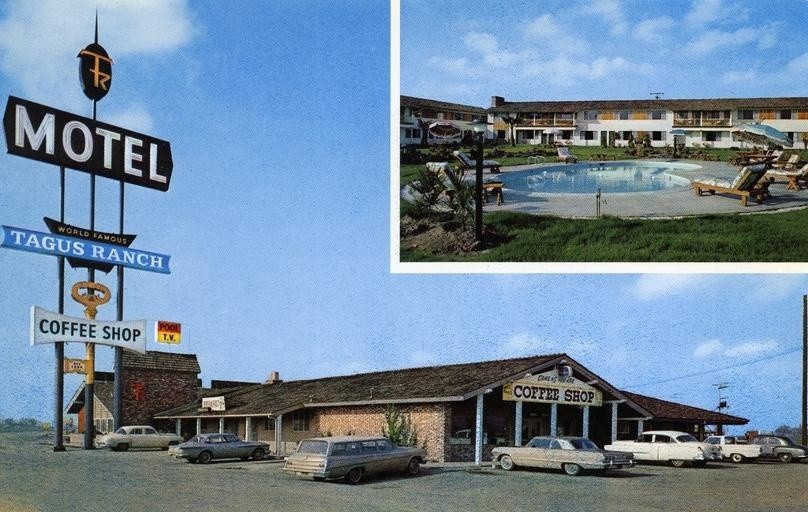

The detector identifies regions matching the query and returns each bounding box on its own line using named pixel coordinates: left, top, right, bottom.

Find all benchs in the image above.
left=587, top=145, right=720, bottom=165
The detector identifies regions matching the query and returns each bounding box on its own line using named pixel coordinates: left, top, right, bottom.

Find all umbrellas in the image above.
left=729, top=123, right=795, bottom=160
left=542, top=124, right=564, bottom=143
left=669, top=127, right=688, bottom=140
left=428, top=117, right=463, bottom=147
left=731, top=127, right=766, bottom=156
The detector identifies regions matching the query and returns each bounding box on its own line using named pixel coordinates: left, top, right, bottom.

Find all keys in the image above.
left=56, top=280, right=113, bottom=388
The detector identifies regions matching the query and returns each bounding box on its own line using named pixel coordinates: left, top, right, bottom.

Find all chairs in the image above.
left=691, top=166, right=770, bottom=206
left=451, top=149, right=501, bottom=173
left=731, top=145, right=806, bottom=191
left=426, top=162, right=505, bottom=206
left=554, top=147, right=577, bottom=164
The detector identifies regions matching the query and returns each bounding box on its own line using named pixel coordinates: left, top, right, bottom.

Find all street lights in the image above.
left=466, top=118, right=497, bottom=246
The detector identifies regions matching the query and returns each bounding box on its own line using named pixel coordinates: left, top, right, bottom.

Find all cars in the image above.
left=170, top=432, right=272, bottom=464
left=491, top=434, right=638, bottom=475
left=452, top=428, right=506, bottom=443
left=96, top=423, right=185, bottom=453
left=604, top=429, right=721, bottom=468
left=748, top=435, right=808, bottom=464
left=283, top=436, right=429, bottom=484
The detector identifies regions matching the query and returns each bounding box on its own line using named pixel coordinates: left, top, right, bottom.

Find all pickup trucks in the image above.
left=703, top=434, right=765, bottom=464
